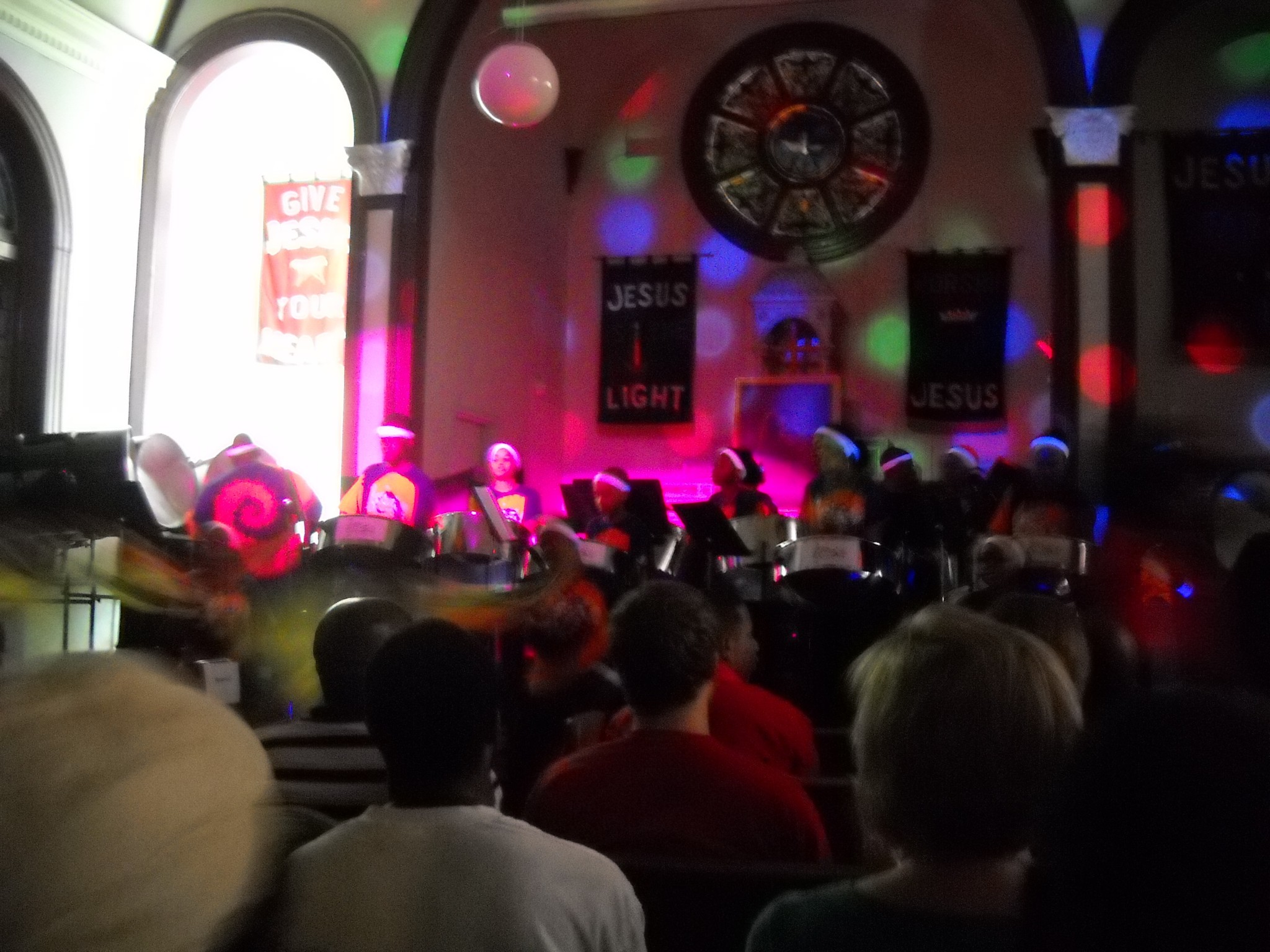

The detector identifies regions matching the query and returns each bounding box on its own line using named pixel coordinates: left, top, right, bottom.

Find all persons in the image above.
left=0, top=420, right=1270, bottom=951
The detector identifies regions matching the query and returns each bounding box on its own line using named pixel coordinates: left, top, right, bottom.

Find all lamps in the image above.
left=472, top=0, right=560, bottom=129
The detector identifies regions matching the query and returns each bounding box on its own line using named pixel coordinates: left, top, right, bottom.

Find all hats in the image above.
left=880, top=448, right=912, bottom=471
left=0, top=650, right=281, bottom=952
left=593, top=467, right=631, bottom=492
left=948, top=444, right=979, bottom=468
left=376, top=415, right=416, bottom=439
left=1028, top=432, right=1069, bottom=459
left=486, top=439, right=522, bottom=471
left=226, top=433, right=258, bottom=456
left=816, top=424, right=861, bottom=462
left=720, top=446, right=761, bottom=485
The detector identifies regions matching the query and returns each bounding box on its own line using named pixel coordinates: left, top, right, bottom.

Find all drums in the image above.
left=519, top=540, right=631, bottom=582
left=716, top=515, right=808, bottom=573
left=305, top=514, right=432, bottom=573
left=433, top=511, right=508, bottom=568
left=967, top=534, right=1097, bottom=573
left=650, top=526, right=692, bottom=580
left=773, top=534, right=882, bottom=586
